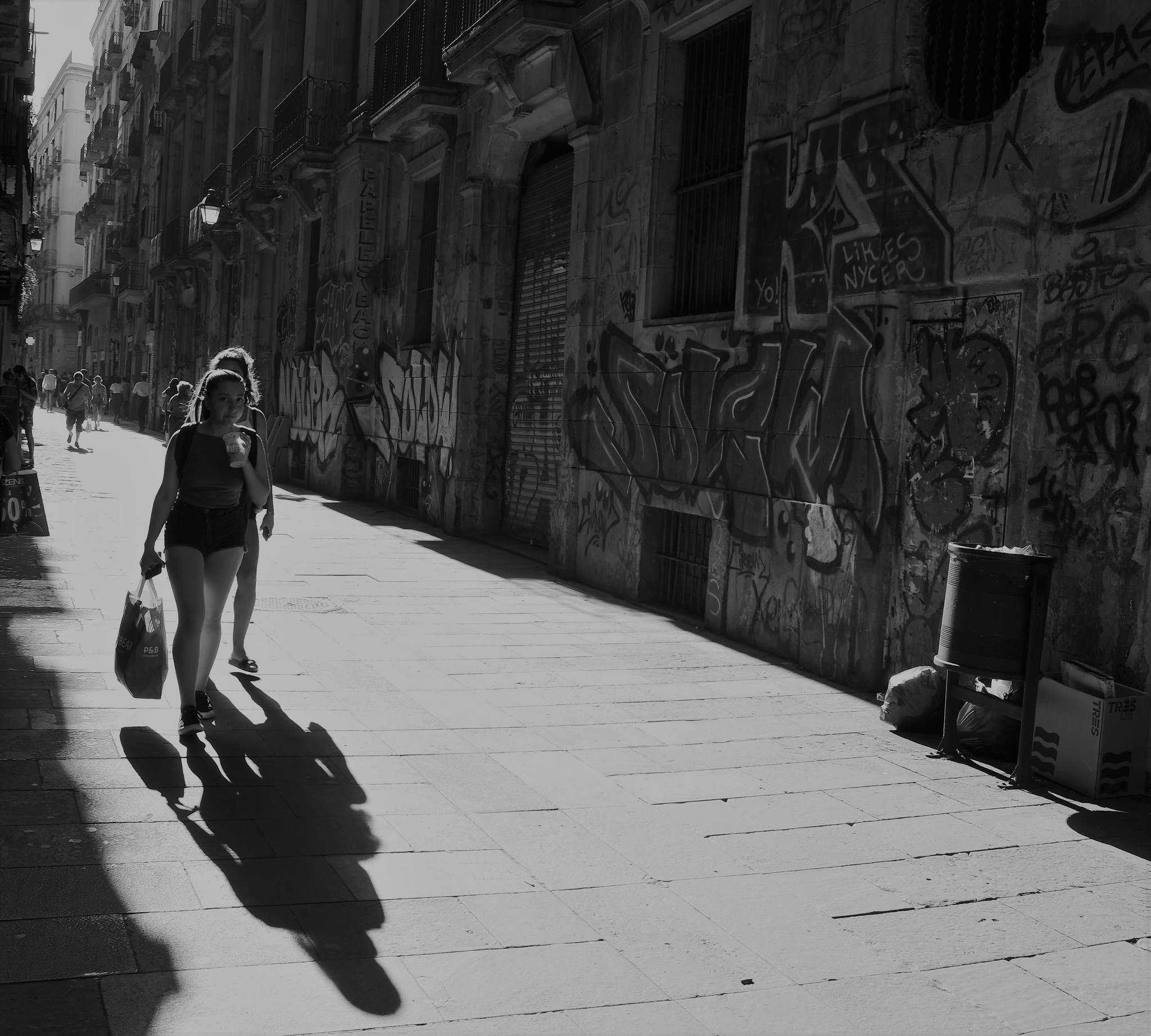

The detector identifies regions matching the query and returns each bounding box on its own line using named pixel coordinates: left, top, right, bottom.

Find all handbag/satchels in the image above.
left=115, top=575, right=169, bottom=700
left=64, top=401, right=69, bottom=410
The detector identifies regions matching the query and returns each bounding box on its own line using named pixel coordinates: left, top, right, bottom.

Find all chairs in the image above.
left=0, top=395, right=20, bottom=446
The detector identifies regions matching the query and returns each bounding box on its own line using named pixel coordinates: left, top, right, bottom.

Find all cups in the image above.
left=223, top=432, right=245, bottom=468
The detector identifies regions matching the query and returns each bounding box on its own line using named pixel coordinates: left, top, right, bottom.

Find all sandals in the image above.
left=162, top=443, right=167, bottom=448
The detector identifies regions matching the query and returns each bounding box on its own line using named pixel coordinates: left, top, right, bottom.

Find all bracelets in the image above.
left=267, top=511, right=274, bottom=515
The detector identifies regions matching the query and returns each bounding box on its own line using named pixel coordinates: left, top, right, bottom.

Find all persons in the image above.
left=186, top=347, right=275, bottom=672
left=0, top=366, right=194, bottom=476
left=139, top=370, right=270, bottom=736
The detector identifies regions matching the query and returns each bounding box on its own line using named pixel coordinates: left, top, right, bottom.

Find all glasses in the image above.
left=14, top=374, right=24, bottom=377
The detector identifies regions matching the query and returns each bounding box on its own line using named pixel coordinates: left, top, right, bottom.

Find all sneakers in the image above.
left=195, top=692, right=214, bottom=720
left=179, top=705, right=204, bottom=736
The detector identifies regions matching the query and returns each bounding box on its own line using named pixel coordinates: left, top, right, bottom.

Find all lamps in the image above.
left=110, top=268, right=124, bottom=290
left=198, top=188, right=240, bottom=263
left=22, top=226, right=46, bottom=265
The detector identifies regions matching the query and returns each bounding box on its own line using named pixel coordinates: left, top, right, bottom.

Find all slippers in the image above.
left=227, top=658, right=259, bottom=673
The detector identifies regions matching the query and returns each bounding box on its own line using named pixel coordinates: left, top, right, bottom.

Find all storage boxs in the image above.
left=1029, top=660, right=1151, bottom=800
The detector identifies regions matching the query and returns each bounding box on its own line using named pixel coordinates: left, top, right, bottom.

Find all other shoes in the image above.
left=74, top=441, right=78, bottom=447
left=86, top=408, right=131, bottom=425
left=94, top=421, right=100, bottom=428
left=40, top=403, right=67, bottom=411
left=67, top=433, right=74, bottom=443
left=139, top=428, right=145, bottom=433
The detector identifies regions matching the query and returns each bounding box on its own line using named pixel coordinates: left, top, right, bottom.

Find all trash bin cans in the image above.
left=930, top=539, right=1056, bottom=684
